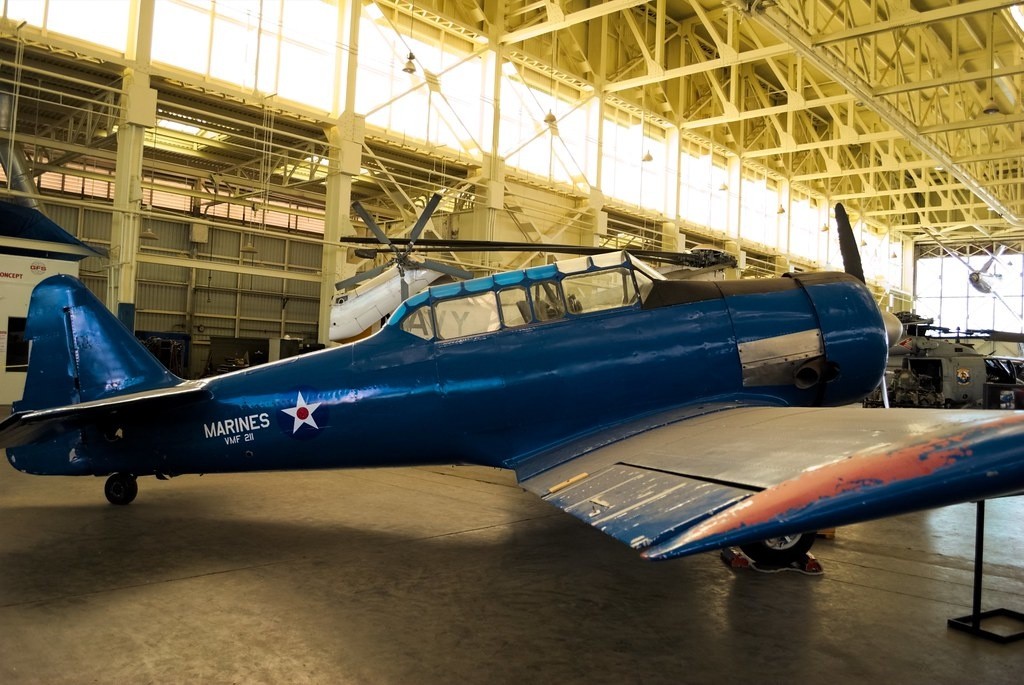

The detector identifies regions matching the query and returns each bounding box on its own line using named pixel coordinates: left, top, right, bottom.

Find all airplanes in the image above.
left=920, top=226, right=1016, bottom=320
left=327, top=192, right=492, bottom=339
left=863, top=310, right=988, bottom=407
left=0, top=202, right=1023, bottom=567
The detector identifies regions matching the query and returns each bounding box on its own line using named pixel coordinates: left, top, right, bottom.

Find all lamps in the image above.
left=860, top=239, right=867, bottom=245
left=643, top=85, right=654, bottom=161
left=891, top=251, right=897, bottom=259
left=776, top=204, right=786, bottom=214
left=403, top=0, right=418, bottom=74
left=542, top=30, right=558, bottom=123
left=717, top=129, right=729, bottom=191
left=821, top=222, right=828, bottom=231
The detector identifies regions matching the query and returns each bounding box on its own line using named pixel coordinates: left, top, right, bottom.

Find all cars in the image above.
left=984, top=356, right=1024, bottom=409
